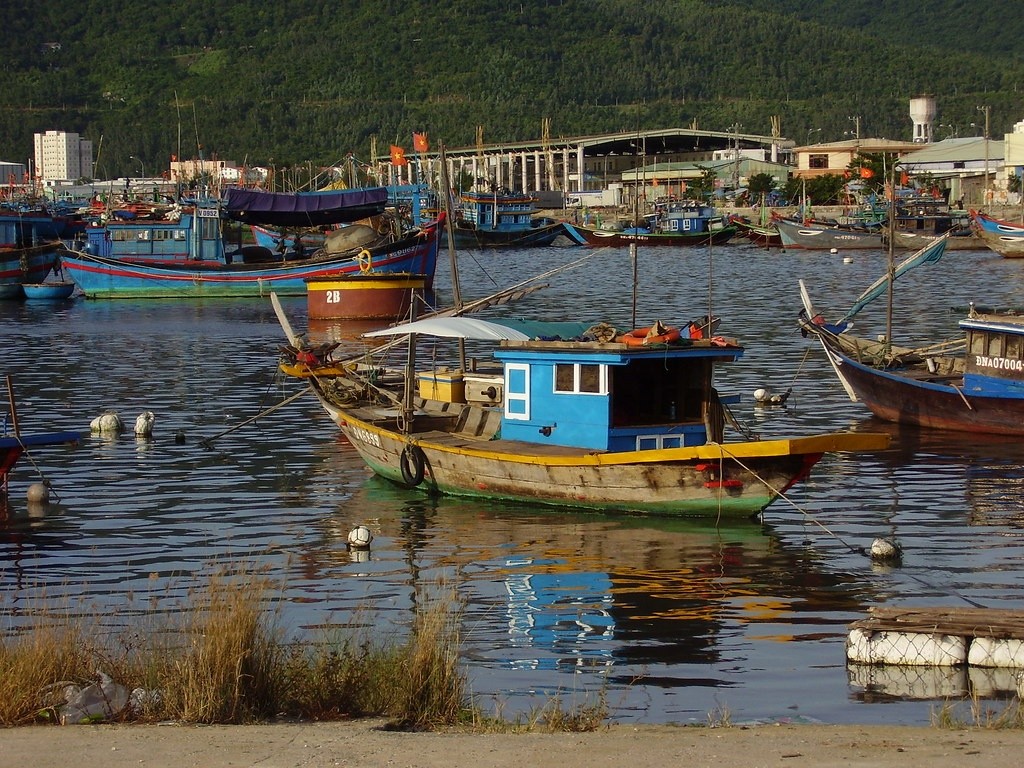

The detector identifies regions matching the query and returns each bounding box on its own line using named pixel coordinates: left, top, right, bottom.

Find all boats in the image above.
left=771, top=217, right=906, bottom=250
left=796, top=226, right=1024, bottom=437
left=0, top=98, right=444, bottom=301
left=438, top=153, right=562, bottom=254
left=970, top=224, right=1024, bottom=258
left=564, top=197, right=738, bottom=249
left=878, top=221, right=989, bottom=250
left=0, top=371, right=83, bottom=490
left=968, top=208, right=1024, bottom=236
left=299, top=268, right=428, bottom=323
left=731, top=216, right=780, bottom=247
left=266, top=143, right=892, bottom=524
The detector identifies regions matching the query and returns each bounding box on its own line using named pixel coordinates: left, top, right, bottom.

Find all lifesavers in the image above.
left=616, top=327, right=679, bottom=346
left=400, top=445, right=425, bottom=485
left=358, top=249, right=372, bottom=272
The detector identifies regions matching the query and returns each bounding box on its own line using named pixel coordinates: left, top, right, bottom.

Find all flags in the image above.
left=901, top=171, right=908, bottom=186
left=414, top=133, right=428, bottom=152
left=861, top=168, right=873, bottom=178
left=390, top=145, right=407, bottom=165
